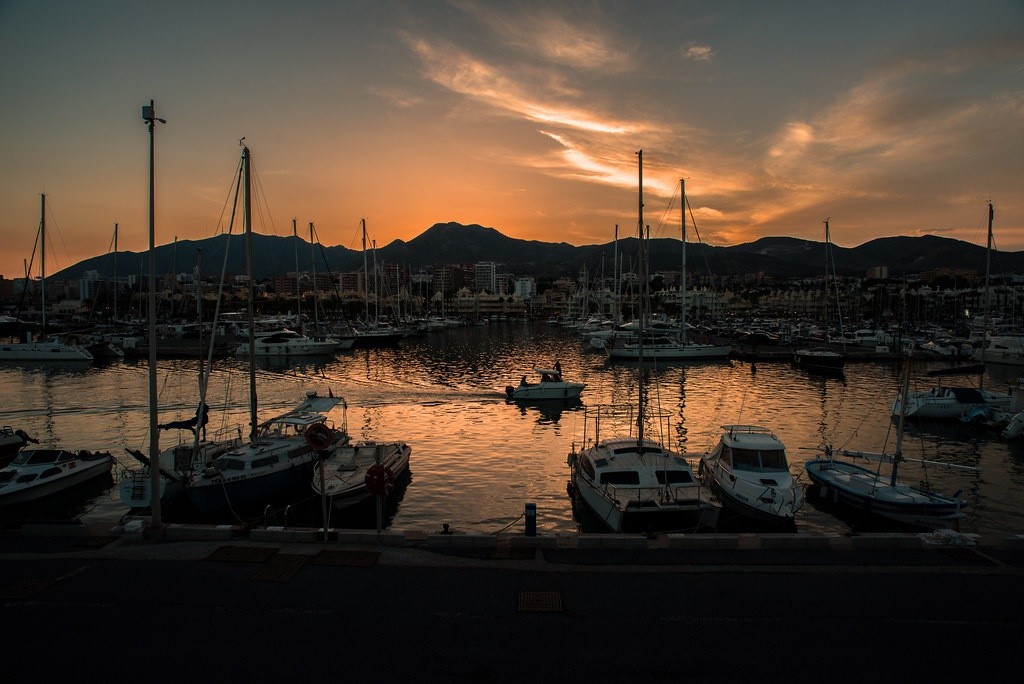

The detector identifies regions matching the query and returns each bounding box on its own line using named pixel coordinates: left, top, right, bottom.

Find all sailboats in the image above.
left=891, top=194, right=1018, bottom=418
left=177, top=136, right=354, bottom=516
left=804, top=338, right=983, bottom=531
left=564, top=180, right=1024, bottom=368
left=0, top=191, right=560, bottom=360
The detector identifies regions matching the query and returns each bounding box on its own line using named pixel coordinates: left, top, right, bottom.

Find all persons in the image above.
left=552, top=361, right=562, bottom=375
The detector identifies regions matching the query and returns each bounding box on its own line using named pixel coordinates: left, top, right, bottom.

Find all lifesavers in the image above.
left=365, top=465, right=395, bottom=496
left=306, top=423, right=332, bottom=450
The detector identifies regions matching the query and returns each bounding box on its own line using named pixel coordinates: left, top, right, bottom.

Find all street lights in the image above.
left=138, top=96, right=175, bottom=528
left=564, top=156, right=723, bottom=533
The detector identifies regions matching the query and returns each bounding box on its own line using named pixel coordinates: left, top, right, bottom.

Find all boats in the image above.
left=16, top=440, right=17, bottom=441
left=0, top=424, right=39, bottom=456
left=924, top=358, right=985, bottom=375
left=119, top=417, right=244, bottom=508
left=0, top=445, right=118, bottom=505
left=503, top=370, right=586, bottom=400
left=308, top=442, right=411, bottom=513
left=699, top=422, right=806, bottom=525
